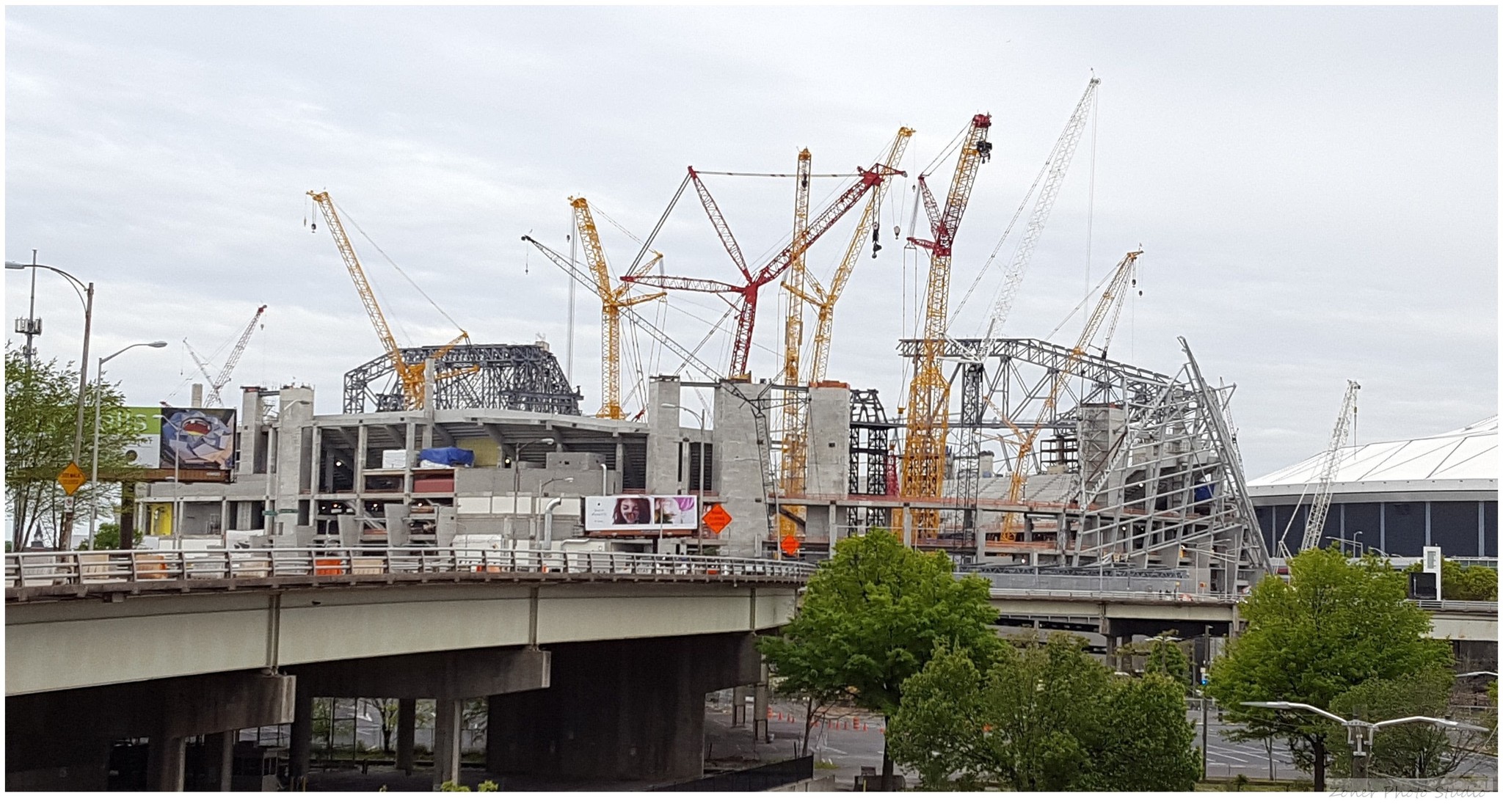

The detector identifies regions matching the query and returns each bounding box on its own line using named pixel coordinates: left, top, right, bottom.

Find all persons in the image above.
left=612, top=498, right=672, bottom=525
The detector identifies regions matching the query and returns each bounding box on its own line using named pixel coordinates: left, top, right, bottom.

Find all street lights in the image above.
left=274, top=399, right=310, bottom=537
left=661, top=403, right=705, bottom=557
left=89, top=340, right=167, bottom=551
left=152, top=414, right=180, bottom=550
left=1353, top=531, right=1363, bottom=558
left=535, top=477, right=574, bottom=572
left=513, top=438, right=554, bottom=572
left=1203, top=624, right=1214, bottom=780
left=1326, top=537, right=1363, bottom=559
left=5, top=259, right=94, bottom=587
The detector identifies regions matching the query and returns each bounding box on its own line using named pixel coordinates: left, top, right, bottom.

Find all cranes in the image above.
left=519, top=73, right=1103, bottom=561
left=304, top=190, right=479, bottom=412
left=1272, top=379, right=1362, bottom=592
left=995, top=248, right=1146, bottom=556
left=159, top=304, right=268, bottom=409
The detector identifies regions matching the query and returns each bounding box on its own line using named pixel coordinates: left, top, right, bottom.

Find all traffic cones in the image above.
left=813, top=717, right=848, bottom=731
left=863, top=722, right=869, bottom=732
left=777, top=712, right=795, bottom=724
